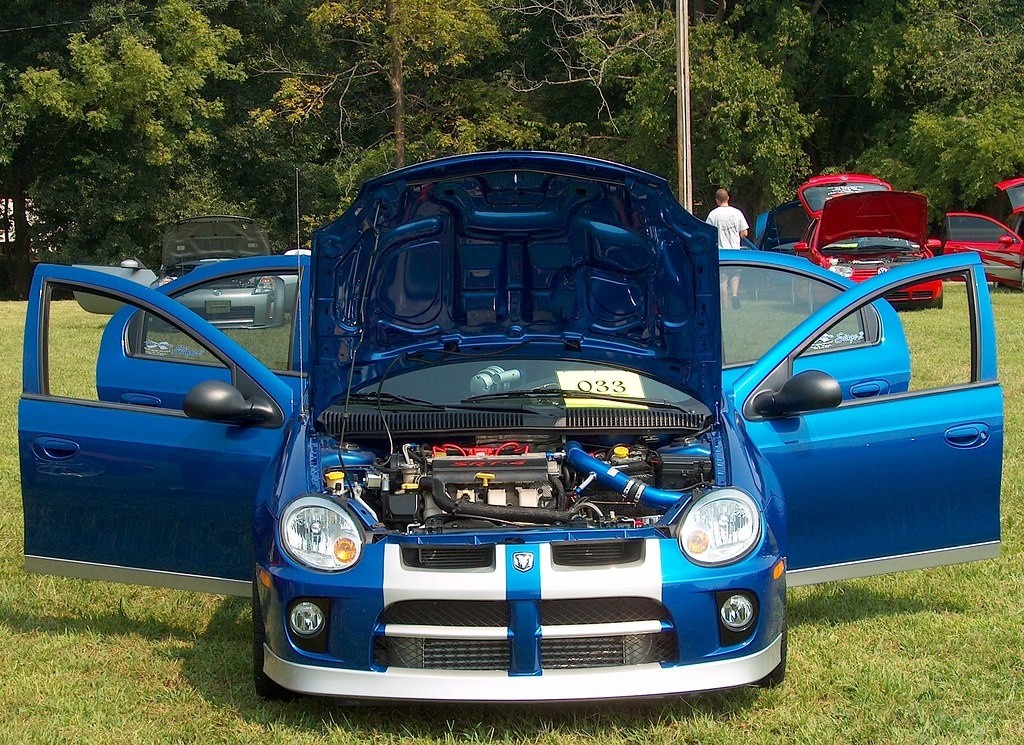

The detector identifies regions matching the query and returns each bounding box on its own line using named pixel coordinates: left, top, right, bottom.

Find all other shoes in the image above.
left=731, top=296, right=741, bottom=310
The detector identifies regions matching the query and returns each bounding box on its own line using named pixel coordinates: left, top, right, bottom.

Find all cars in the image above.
left=19, top=148, right=1007, bottom=708
left=794, top=174, right=946, bottom=311
left=719, top=197, right=811, bottom=257
left=69, top=216, right=311, bottom=331
left=943, top=178, right=1024, bottom=292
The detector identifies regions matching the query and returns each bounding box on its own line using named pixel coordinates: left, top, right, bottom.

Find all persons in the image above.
left=705, top=187, right=749, bottom=312
